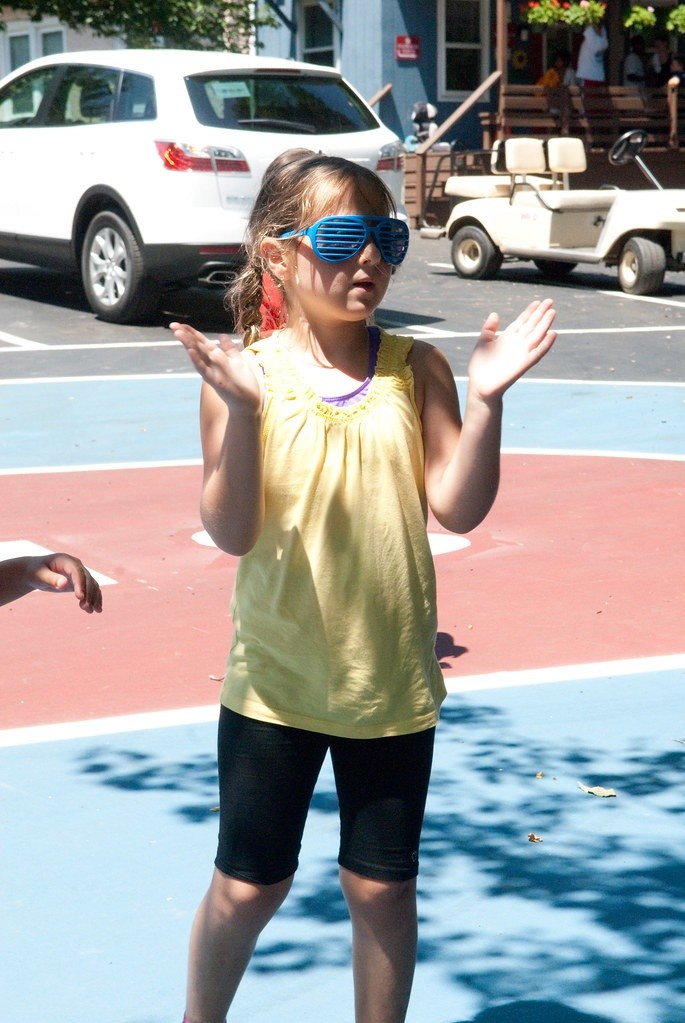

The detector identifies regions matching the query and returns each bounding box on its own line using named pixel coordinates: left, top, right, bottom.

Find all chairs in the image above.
left=79, top=81, right=111, bottom=123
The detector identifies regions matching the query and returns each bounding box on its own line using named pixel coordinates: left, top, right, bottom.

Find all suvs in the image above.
left=0, top=48, right=411, bottom=327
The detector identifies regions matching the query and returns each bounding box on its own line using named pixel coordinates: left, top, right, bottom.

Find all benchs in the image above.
left=478, top=86, right=669, bottom=150
left=444, top=136, right=624, bottom=210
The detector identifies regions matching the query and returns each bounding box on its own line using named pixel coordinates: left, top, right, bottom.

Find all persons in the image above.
left=169, top=149, right=559, bottom=1023
left=624, top=34, right=685, bottom=149
left=534, top=50, right=580, bottom=133
left=0, top=553, right=103, bottom=614
left=575, top=21, right=609, bottom=115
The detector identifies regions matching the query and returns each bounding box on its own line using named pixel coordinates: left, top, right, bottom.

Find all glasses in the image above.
left=277, top=214, right=411, bottom=267
